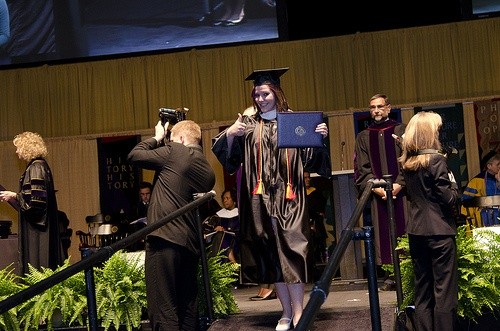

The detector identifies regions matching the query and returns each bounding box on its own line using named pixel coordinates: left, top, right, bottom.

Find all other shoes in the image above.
left=249, top=289, right=277, bottom=301
left=275, top=314, right=299, bottom=331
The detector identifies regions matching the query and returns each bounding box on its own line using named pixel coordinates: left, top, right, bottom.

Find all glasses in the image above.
left=368, top=104, right=388, bottom=110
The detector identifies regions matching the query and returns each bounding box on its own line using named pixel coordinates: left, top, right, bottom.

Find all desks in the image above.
left=0, top=234, right=20, bottom=282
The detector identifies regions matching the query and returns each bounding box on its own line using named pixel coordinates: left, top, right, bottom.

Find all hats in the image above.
left=244, top=67, right=290, bottom=86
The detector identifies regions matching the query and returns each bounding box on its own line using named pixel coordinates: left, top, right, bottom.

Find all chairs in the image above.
left=75, top=212, right=133, bottom=261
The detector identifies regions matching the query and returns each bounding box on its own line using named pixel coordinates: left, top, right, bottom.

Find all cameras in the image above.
left=159, top=109, right=185, bottom=147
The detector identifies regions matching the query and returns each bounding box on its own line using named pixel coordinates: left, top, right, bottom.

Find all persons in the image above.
left=397, top=111, right=459, bottom=331
left=202, top=67, right=332, bottom=331
left=461, top=151, right=500, bottom=237
left=125, top=182, right=153, bottom=234
left=353, top=94, right=408, bottom=292
left=127, top=120, right=216, bottom=331
left=0, top=132, right=65, bottom=283
